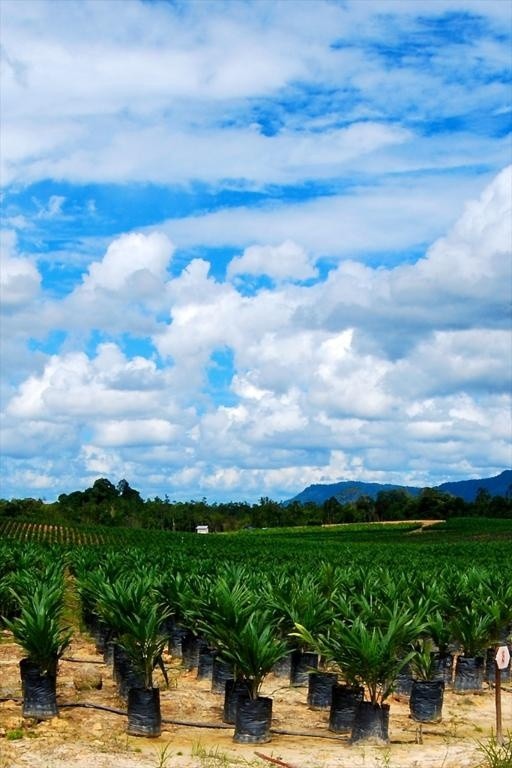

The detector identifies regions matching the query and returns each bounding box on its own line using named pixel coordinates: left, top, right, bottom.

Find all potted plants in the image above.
left=195, top=609, right=297, bottom=743
left=396, top=597, right=464, bottom=723
left=85, top=578, right=178, bottom=740
left=1, top=518, right=511, bottom=736
left=12, top=587, right=75, bottom=721
left=287, top=603, right=411, bottom=744
